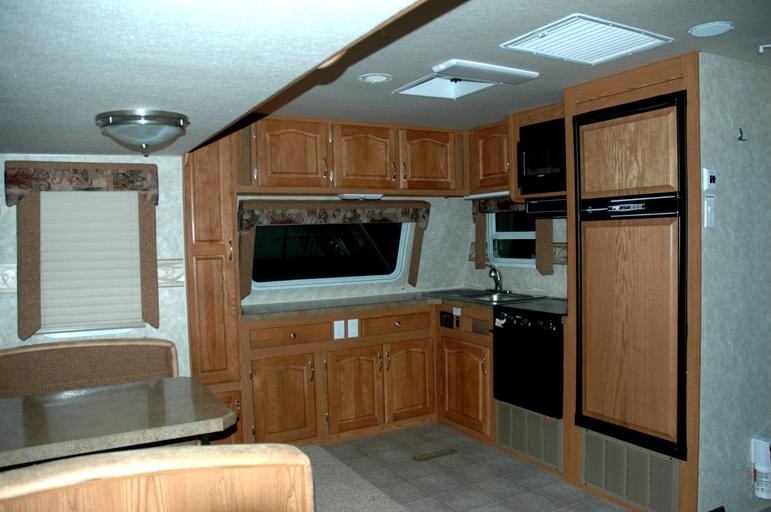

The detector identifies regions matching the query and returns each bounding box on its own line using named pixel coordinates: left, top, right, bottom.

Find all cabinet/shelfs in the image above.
left=180, top=126, right=246, bottom=446
left=440, top=302, right=495, bottom=446
left=246, top=118, right=333, bottom=189
left=457, top=121, right=514, bottom=198
left=332, top=303, right=438, bottom=441
left=335, top=120, right=456, bottom=191
left=246, top=310, right=331, bottom=446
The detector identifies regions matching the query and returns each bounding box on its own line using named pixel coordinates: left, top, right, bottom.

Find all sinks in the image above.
left=467, top=293, right=534, bottom=304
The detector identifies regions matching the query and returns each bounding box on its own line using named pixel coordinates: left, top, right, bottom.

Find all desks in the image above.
left=1, top=374, right=240, bottom=471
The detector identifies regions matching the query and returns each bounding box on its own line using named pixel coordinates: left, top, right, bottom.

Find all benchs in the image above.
left=1, top=339, right=180, bottom=388
left=0, top=442, right=316, bottom=512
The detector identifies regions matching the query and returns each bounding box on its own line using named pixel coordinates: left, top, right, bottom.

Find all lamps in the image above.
left=94, top=108, right=192, bottom=158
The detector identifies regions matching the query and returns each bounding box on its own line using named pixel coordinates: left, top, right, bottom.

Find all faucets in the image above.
left=489, top=268, right=501, bottom=291
